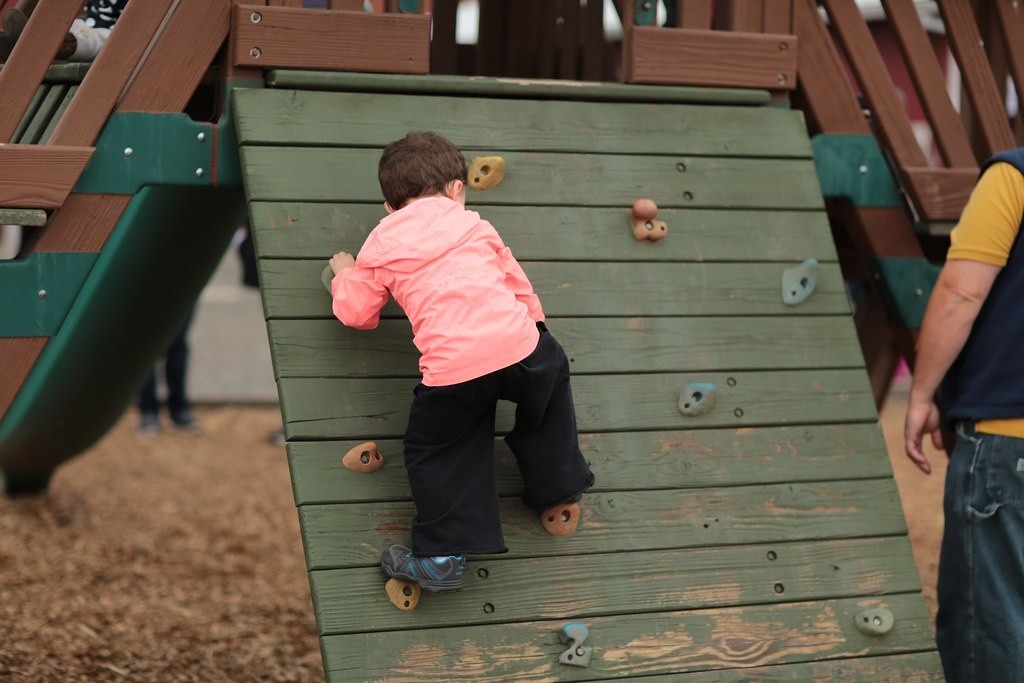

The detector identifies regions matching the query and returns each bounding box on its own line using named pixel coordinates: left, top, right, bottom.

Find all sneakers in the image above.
left=380, top=543, right=465, bottom=593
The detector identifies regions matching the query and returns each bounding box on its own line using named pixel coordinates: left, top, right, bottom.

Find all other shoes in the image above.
left=138, top=410, right=159, bottom=440
left=178, top=418, right=198, bottom=433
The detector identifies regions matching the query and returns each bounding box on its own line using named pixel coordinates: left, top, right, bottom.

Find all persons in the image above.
left=0, top=0, right=127, bottom=63
left=903, top=146, right=1024, bottom=683
left=332, top=131, right=597, bottom=594
left=137, top=331, right=200, bottom=434
